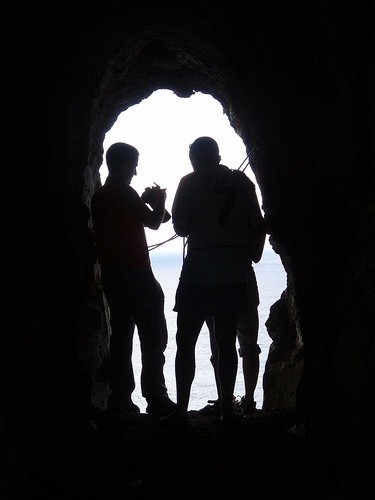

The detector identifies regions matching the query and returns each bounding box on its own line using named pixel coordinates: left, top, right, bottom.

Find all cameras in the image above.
left=139, top=185, right=171, bottom=224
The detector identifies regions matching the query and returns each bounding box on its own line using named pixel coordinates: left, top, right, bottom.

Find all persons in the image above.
left=201, top=188, right=267, bottom=410
left=88, top=142, right=180, bottom=417
left=173, top=135, right=251, bottom=415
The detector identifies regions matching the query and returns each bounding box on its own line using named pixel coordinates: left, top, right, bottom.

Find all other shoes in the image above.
left=214, top=406, right=234, bottom=425
left=147, top=399, right=176, bottom=417
left=108, top=402, right=139, bottom=416
left=234, top=400, right=258, bottom=416
left=205, top=399, right=233, bottom=415
left=176, top=403, right=188, bottom=419
